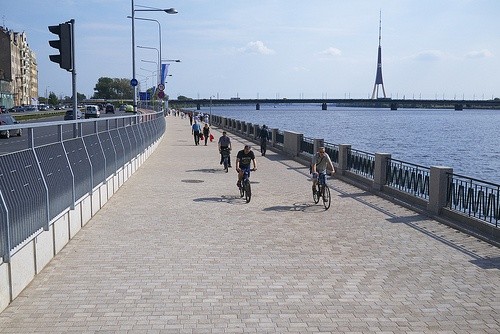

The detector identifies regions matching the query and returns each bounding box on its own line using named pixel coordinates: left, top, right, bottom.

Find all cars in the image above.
left=0, top=100, right=105, bottom=115
left=119, top=103, right=128, bottom=111
left=125, top=104, right=134, bottom=113
left=63, top=109, right=85, bottom=120
left=0, top=114, right=23, bottom=139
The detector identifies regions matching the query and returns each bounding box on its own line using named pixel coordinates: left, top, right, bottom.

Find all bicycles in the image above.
left=236, top=168, right=257, bottom=203
left=312, top=171, right=333, bottom=210
left=219, top=147, right=233, bottom=173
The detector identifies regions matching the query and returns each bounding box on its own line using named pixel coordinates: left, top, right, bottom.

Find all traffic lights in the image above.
left=48, top=23, right=72, bottom=71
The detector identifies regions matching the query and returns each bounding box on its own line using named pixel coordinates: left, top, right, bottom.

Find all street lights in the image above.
left=131, top=0, right=178, bottom=119
left=127, top=15, right=182, bottom=113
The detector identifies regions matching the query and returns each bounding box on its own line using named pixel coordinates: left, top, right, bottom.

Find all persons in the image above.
left=218, top=131, right=232, bottom=167
left=257, top=125, right=269, bottom=156
left=236, top=145, right=257, bottom=196
left=192, top=120, right=204, bottom=146
left=311, top=147, right=335, bottom=202
left=203, top=124, right=214, bottom=146
left=204, top=113, right=209, bottom=124
left=174, top=110, right=179, bottom=117
left=190, top=115, right=193, bottom=125
left=194, top=114, right=200, bottom=123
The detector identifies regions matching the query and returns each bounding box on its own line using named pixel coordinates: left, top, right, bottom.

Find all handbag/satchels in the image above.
left=310, top=166, right=312, bottom=174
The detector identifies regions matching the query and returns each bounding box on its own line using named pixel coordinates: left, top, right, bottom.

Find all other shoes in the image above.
left=228, top=165, right=231, bottom=167
left=237, top=183, right=241, bottom=187
left=312, top=185, right=316, bottom=191
left=324, top=198, right=328, bottom=202
left=220, top=161, right=223, bottom=164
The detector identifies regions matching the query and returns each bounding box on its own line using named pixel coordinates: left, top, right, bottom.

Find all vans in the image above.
left=105, top=104, right=115, bottom=114
left=85, top=104, right=101, bottom=119
